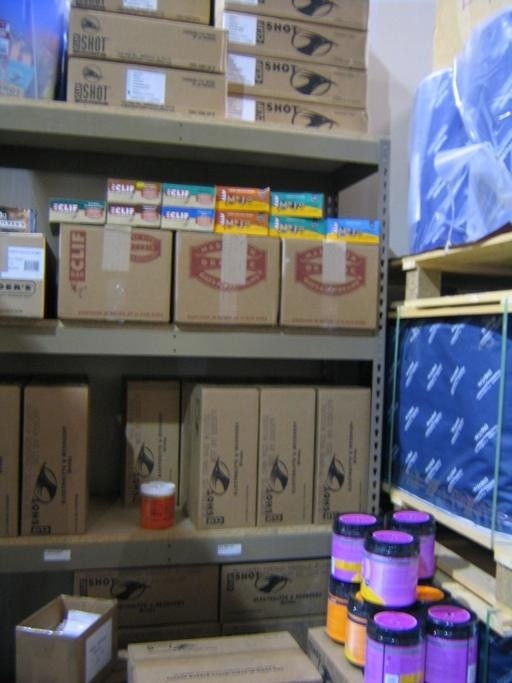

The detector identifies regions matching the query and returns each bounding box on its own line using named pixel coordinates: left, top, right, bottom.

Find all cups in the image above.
left=140, top=481, right=176, bottom=530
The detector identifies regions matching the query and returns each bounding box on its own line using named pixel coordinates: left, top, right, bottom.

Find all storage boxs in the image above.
left=1, top=374, right=373, bottom=528
left=125, top=628, right=324, bottom=682
left=305, top=621, right=367, bottom=683
left=1, top=175, right=381, bottom=335
left=67, top=0, right=369, bottom=137
left=15, top=594, right=122, bottom=680
left=72, top=557, right=341, bottom=652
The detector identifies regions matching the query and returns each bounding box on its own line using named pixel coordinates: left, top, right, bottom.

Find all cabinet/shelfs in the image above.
left=1, top=97, right=393, bottom=581
left=382, top=228, right=511, bottom=638
